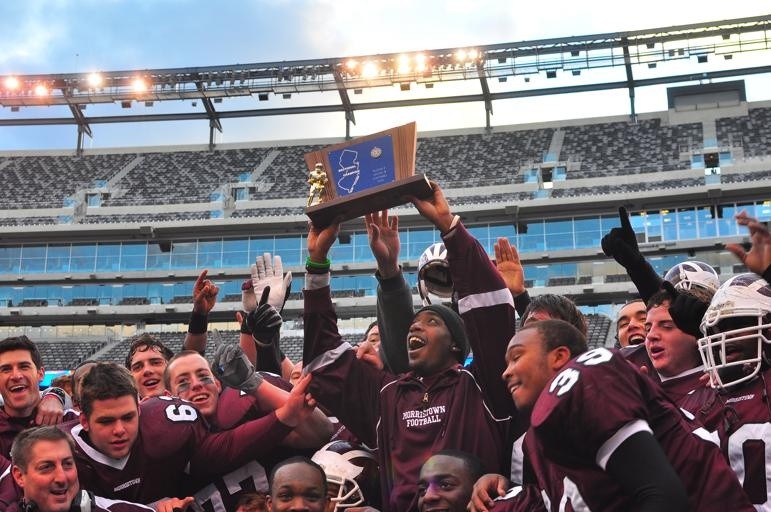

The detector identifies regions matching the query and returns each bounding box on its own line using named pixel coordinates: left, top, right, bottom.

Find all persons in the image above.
left=418, top=448, right=486, bottom=511
left=1, top=253, right=383, bottom=511
left=364, top=209, right=531, bottom=472
left=301, top=183, right=515, bottom=510
left=481, top=293, right=587, bottom=466
left=601, top=207, right=769, bottom=511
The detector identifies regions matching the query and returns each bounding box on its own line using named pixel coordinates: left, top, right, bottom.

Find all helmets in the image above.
left=664, top=260, right=720, bottom=300
left=696, top=273, right=770, bottom=390
left=309, top=438, right=381, bottom=512
left=416, top=237, right=463, bottom=309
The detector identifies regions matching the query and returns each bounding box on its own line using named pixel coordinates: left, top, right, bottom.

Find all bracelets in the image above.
left=304, top=265, right=330, bottom=275
left=241, top=313, right=252, bottom=333
left=189, top=309, right=207, bottom=336
left=306, top=258, right=331, bottom=268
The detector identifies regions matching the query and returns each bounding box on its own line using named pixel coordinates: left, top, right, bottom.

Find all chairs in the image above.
left=0, top=104, right=771, bottom=230
left=0, top=270, right=646, bottom=375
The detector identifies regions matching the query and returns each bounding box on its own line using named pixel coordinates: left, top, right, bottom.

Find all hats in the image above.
left=417, top=300, right=475, bottom=369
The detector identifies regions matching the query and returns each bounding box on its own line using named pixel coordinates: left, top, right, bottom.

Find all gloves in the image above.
left=240, top=248, right=293, bottom=372
left=601, top=206, right=664, bottom=304
left=208, top=327, right=264, bottom=394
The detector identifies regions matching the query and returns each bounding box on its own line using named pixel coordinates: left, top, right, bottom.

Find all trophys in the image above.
left=300, top=121, right=434, bottom=231
